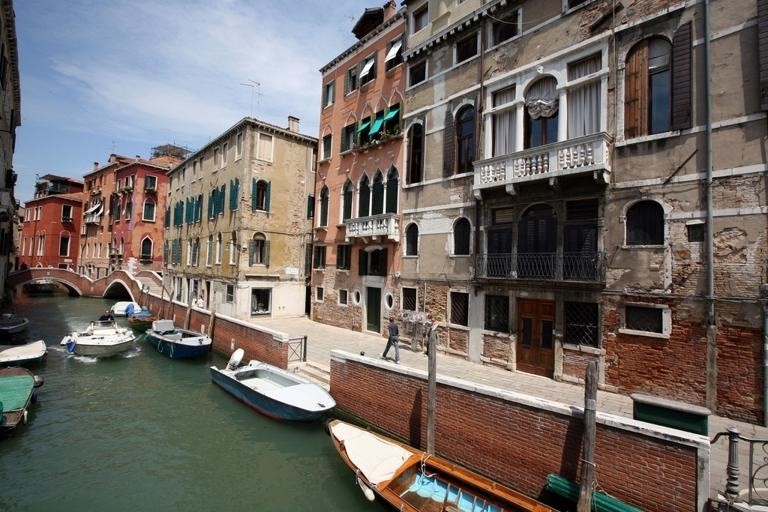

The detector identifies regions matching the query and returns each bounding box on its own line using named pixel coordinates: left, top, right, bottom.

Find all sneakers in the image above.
left=380, top=356, right=400, bottom=364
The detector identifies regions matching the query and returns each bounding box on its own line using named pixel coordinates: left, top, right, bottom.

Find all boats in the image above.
left=210, top=360, right=335, bottom=421
left=0, top=313, right=29, bottom=334
left=24, top=280, right=56, bottom=293
left=325, top=417, right=563, bottom=512
left=0, top=366, right=44, bottom=433
left=73, top=320, right=134, bottom=357
left=0, top=340, right=48, bottom=362
left=110, top=301, right=161, bottom=332
left=145, top=320, right=213, bottom=359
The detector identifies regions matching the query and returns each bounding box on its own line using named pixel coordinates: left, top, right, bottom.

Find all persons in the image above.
left=426, top=315, right=439, bottom=356
left=98, top=310, right=116, bottom=326
left=195, top=295, right=205, bottom=308
left=381, top=316, right=400, bottom=363
